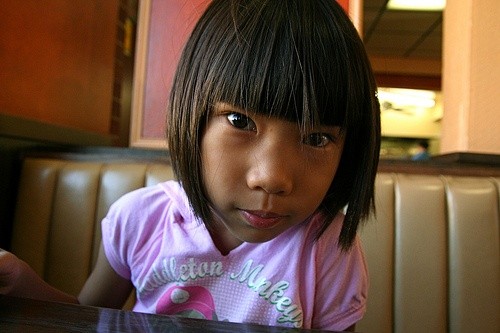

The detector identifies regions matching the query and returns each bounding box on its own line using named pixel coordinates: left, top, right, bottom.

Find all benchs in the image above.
left=22, top=143, right=499, bottom=332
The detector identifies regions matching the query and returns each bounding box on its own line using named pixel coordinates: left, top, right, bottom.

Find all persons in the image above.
left=1, top=0, right=383, bottom=333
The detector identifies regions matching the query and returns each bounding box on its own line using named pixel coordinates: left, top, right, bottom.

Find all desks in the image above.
left=0, top=295, right=345, bottom=332
